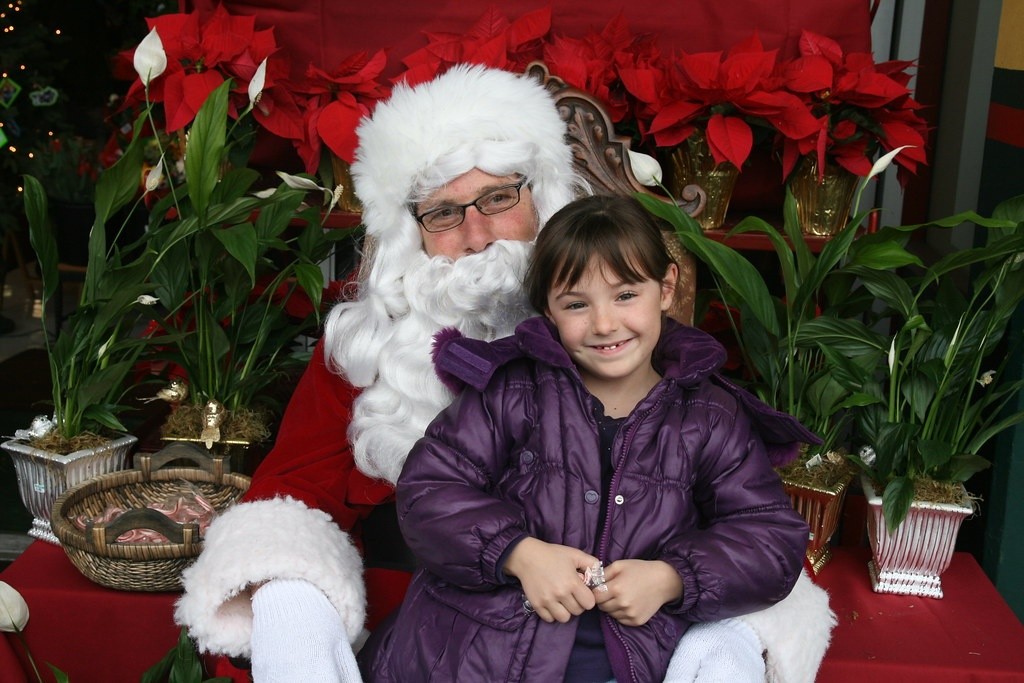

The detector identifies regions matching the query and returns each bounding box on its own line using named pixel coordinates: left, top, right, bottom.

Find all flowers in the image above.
left=0, top=0, right=1024, bottom=539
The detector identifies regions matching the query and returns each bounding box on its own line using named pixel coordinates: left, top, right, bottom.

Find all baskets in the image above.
left=50, top=456, right=252, bottom=592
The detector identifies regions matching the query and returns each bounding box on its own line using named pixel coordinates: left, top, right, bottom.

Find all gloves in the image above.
left=250, top=578, right=362, bottom=683
left=662, top=617, right=766, bottom=683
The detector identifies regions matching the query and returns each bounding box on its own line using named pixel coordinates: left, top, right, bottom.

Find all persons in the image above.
left=367, top=193, right=810, bottom=683
left=172, top=63, right=836, bottom=683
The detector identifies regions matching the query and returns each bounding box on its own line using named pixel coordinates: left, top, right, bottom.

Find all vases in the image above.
left=0, top=429, right=139, bottom=547
left=666, top=122, right=740, bottom=230
left=329, top=152, right=363, bottom=213
left=779, top=469, right=858, bottom=573
left=775, top=147, right=876, bottom=237
left=858, top=471, right=974, bottom=600
left=160, top=436, right=254, bottom=475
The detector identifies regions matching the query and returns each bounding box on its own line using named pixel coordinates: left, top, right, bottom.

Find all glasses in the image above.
left=410, top=173, right=526, bottom=233
